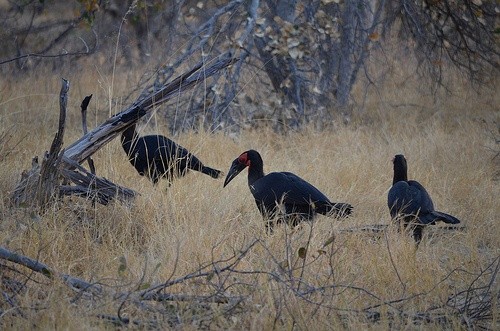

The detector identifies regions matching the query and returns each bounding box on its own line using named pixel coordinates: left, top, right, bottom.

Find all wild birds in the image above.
left=120, top=124, right=223, bottom=191
left=388, top=154, right=460, bottom=244
left=222, top=150, right=354, bottom=236
